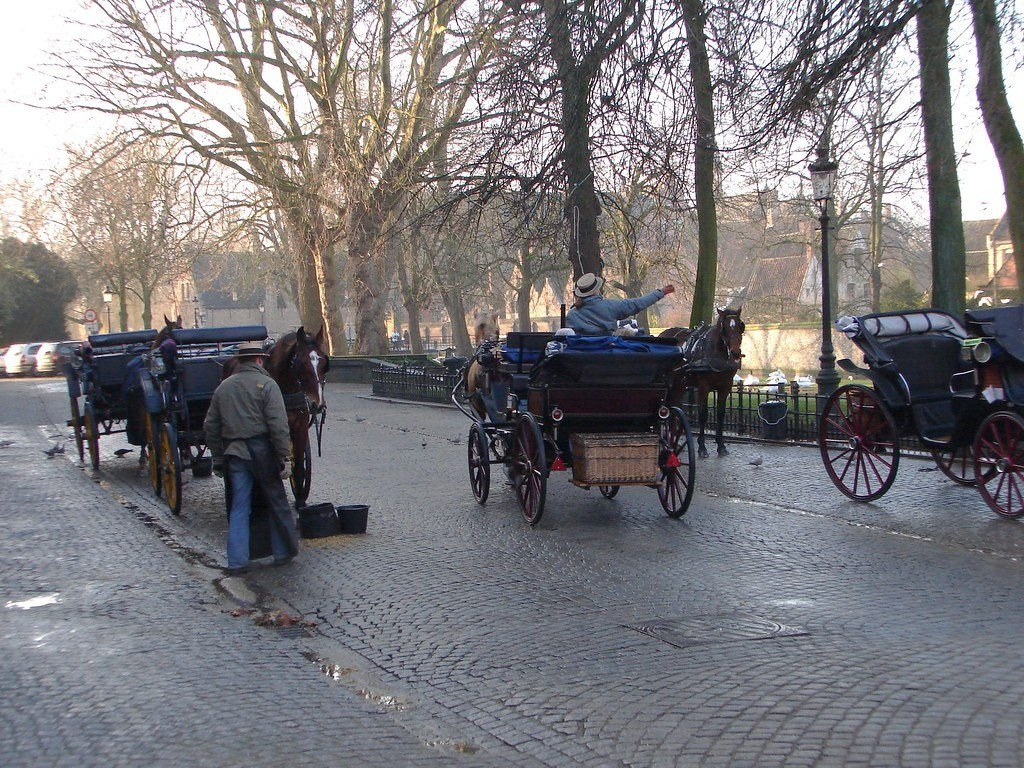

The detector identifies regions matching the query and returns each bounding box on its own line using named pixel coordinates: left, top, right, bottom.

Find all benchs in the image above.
left=172, top=326, right=267, bottom=408
left=994, top=351, right=1024, bottom=407
left=88, top=329, right=159, bottom=388
left=881, top=335, right=986, bottom=403
left=506, top=331, right=686, bottom=393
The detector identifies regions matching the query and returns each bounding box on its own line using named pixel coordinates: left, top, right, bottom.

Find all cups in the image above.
left=559, top=342, right=567, bottom=352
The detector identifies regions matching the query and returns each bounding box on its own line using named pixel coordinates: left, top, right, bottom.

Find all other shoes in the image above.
left=229, top=559, right=262, bottom=574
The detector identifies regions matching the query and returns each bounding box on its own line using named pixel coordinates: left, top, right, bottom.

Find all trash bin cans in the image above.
left=443, top=356, right=468, bottom=398
left=759, top=400, right=789, bottom=439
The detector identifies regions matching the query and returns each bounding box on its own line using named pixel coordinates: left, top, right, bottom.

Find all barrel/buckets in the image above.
left=192, top=457, right=212, bottom=476
left=336, top=505, right=370, bottom=534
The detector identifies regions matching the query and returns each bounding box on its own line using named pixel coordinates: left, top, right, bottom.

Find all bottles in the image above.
left=507, top=394, right=517, bottom=409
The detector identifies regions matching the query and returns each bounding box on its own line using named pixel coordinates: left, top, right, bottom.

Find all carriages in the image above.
left=61, top=313, right=186, bottom=471
left=449, top=302, right=695, bottom=525
left=130, top=320, right=332, bottom=515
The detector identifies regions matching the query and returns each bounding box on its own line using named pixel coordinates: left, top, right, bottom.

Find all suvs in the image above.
left=32, top=340, right=87, bottom=378
left=4, top=342, right=45, bottom=378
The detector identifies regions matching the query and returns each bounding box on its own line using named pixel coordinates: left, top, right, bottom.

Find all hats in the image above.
left=229, top=342, right=271, bottom=356
left=575, top=274, right=604, bottom=298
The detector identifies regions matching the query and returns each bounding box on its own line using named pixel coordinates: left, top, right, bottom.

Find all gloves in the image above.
left=280, top=458, right=292, bottom=479
left=661, top=286, right=674, bottom=295
left=213, top=458, right=225, bottom=479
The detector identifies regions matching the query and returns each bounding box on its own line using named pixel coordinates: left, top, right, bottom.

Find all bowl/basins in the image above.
left=555, top=328, right=576, bottom=335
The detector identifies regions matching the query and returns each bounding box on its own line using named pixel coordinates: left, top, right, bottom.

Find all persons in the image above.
left=392, top=330, right=400, bottom=351
left=565, top=273, right=674, bottom=335
left=403, top=330, right=409, bottom=350
left=203, top=343, right=299, bottom=573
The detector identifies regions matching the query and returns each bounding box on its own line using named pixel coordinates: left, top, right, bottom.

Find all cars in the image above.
left=0, top=347, right=9, bottom=377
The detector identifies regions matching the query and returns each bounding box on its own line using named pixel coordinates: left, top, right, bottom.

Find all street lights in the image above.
left=809, top=147, right=843, bottom=440
left=102, top=285, right=114, bottom=333
left=191, top=296, right=199, bottom=329
left=259, top=301, right=265, bottom=325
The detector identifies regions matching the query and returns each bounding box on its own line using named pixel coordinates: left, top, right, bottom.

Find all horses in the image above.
left=462, top=342, right=505, bottom=429
left=265, top=324, right=330, bottom=512
left=658, top=305, right=745, bottom=458
left=125, top=314, right=183, bottom=476
